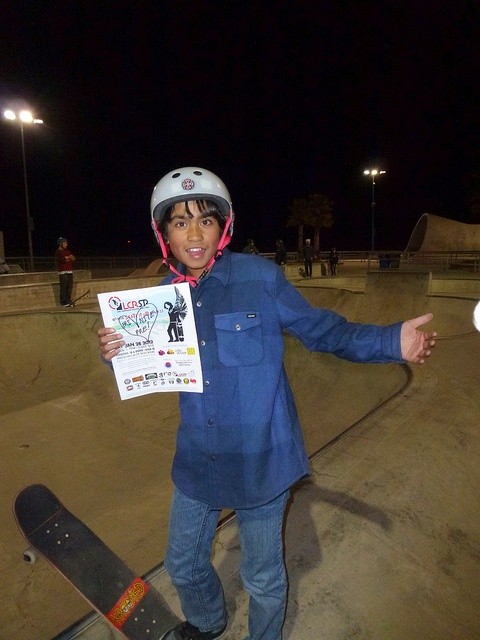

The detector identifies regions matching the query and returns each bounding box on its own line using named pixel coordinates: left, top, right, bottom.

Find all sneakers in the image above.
left=71, top=303, right=77, bottom=306
left=160, top=619, right=228, bottom=640
left=64, top=304, right=73, bottom=308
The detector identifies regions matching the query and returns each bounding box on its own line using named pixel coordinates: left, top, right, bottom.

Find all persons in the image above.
left=94, top=169, right=438, bottom=639
left=243, top=240, right=259, bottom=256
left=327, top=248, right=337, bottom=276
left=302, top=236, right=313, bottom=278
left=272, top=238, right=287, bottom=273
left=53, top=237, right=78, bottom=308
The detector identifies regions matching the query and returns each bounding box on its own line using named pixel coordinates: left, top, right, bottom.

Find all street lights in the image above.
left=363, top=169, right=387, bottom=251
left=4, top=109, right=45, bottom=272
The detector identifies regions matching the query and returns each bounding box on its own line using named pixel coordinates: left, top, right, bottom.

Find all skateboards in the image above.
left=11, top=482, right=184, bottom=639
left=65, top=287, right=91, bottom=310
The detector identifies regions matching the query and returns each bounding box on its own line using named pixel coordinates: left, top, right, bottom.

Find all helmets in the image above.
left=57, top=237, right=67, bottom=246
left=150, top=165, right=235, bottom=245
